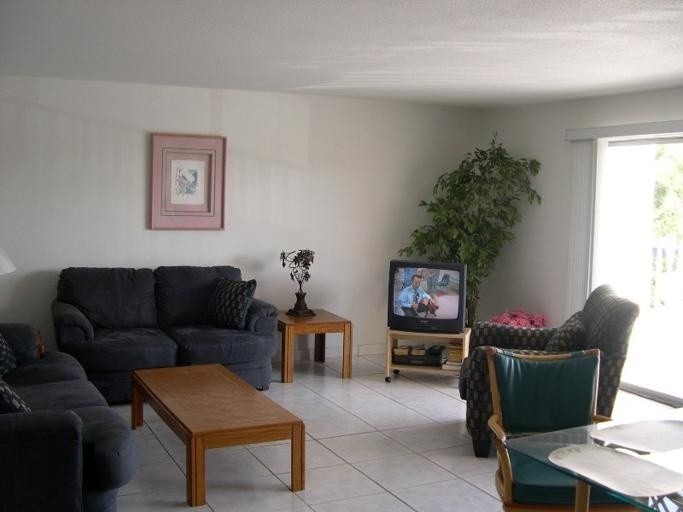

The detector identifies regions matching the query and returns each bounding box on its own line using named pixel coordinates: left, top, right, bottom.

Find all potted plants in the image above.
left=281, top=249, right=316, bottom=318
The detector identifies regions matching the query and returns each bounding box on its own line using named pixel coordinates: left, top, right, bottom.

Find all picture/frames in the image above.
left=149, top=133, right=227, bottom=231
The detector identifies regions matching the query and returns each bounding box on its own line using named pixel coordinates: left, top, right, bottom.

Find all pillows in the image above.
left=204, top=277, right=256, bottom=329
left=0, top=335, right=17, bottom=376
left=544, top=312, right=590, bottom=351
left=0, top=379, right=31, bottom=414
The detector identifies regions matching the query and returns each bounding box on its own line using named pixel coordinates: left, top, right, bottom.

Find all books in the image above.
left=409, top=344, right=437, bottom=355
left=441, top=339, right=462, bottom=372
left=392, top=344, right=411, bottom=355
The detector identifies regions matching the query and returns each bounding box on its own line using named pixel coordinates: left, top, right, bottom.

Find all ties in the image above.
left=414, top=290, right=419, bottom=303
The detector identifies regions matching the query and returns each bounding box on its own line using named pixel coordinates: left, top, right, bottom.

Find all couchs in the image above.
left=0, top=323, right=137, bottom=512
left=50, top=266, right=279, bottom=405
left=459, top=285, right=638, bottom=458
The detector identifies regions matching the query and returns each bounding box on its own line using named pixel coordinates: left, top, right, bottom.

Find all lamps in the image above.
left=0, top=249, right=17, bottom=275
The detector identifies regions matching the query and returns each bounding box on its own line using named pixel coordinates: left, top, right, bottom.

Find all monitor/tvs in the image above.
left=386, top=259, right=468, bottom=334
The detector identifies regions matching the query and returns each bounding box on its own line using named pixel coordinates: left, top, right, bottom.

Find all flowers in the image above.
left=489, top=309, right=551, bottom=328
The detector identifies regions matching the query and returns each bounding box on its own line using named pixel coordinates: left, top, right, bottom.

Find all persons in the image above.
left=399, top=274, right=434, bottom=319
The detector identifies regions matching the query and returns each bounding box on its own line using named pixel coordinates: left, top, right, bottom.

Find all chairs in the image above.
left=486, top=346, right=651, bottom=512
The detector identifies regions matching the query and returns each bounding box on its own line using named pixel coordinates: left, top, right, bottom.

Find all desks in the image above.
left=385, top=328, right=471, bottom=382
left=504, top=406, right=683, bottom=512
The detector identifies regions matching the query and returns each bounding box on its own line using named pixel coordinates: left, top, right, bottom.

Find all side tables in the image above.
left=277, top=309, right=353, bottom=383
